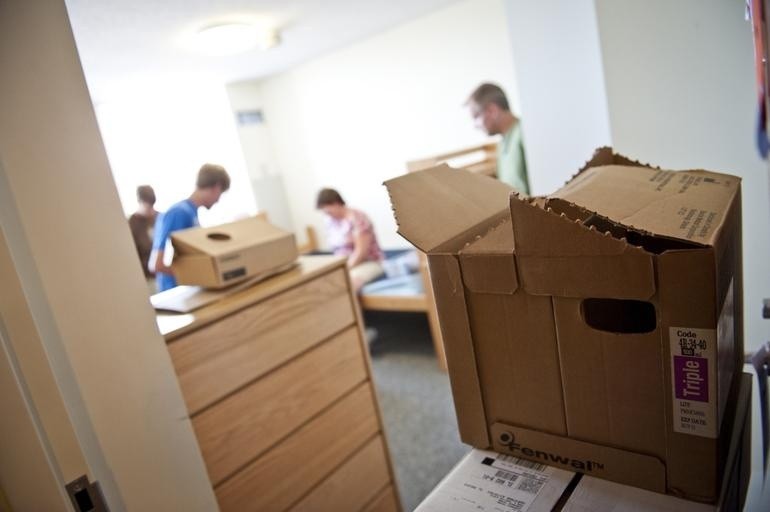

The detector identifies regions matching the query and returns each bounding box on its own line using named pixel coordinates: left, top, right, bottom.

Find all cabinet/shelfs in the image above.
left=149, top=255, right=401, bottom=510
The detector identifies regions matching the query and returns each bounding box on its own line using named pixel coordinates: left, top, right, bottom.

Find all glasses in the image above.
left=470, top=111, right=480, bottom=119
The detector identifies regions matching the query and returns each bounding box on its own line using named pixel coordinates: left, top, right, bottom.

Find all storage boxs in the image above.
left=405, top=445, right=710, bottom=511
left=382, top=142, right=745, bottom=505
left=172, top=217, right=297, bottom=288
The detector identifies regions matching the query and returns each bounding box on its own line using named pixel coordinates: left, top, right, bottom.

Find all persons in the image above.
left=316, top=189, right=386, bottom=296
left=462, top=83, right=531, bottom=197
left=129, top=186, right=160, bottom=280
left=148, top=163, right=230, bottom=292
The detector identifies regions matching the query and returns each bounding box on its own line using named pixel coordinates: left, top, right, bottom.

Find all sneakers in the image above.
left=366, top=328, right=378, bottom=343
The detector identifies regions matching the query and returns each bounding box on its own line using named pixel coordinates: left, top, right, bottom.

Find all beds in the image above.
left=256, top=143, right=497, bottom=372
